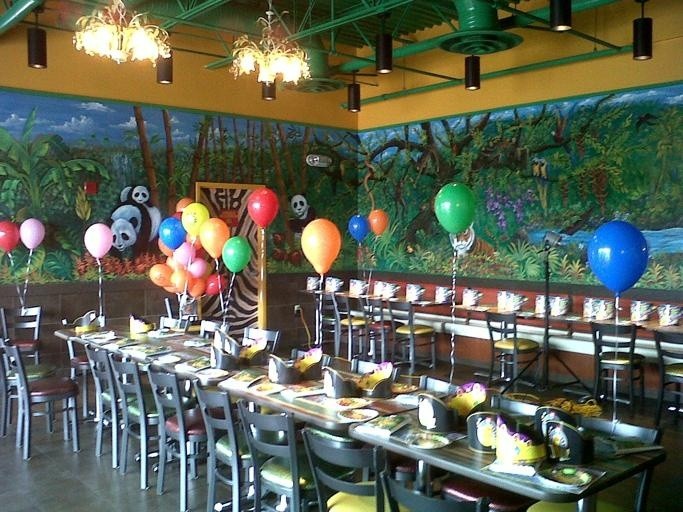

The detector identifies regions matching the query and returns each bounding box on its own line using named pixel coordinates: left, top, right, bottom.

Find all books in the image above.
left=347, top=215, right=368, bottom=244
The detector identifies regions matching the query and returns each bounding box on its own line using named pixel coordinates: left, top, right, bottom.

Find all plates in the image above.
left=63, top=310, right=592, bottom=489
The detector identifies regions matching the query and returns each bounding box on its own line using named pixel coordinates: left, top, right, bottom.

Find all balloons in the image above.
left=83, top=223, right=113, bottom=259
left=18, top=218, right=46, bottom=250
left=146, top=197, right=253, bottom=301
left=246, top=187, right=280, bottom=229
left=586, top=220, right=650, bottom=298
left=300, top=218, right=343, bottom=276
left=0, top=221, right=20, bottom=253
left=367, top=209, right=389, bottom=236
left=434, top=181, right=478, bottom=234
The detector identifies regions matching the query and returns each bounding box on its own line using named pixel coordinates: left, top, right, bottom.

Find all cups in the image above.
left=306, top=275, right=681, bottom=328
